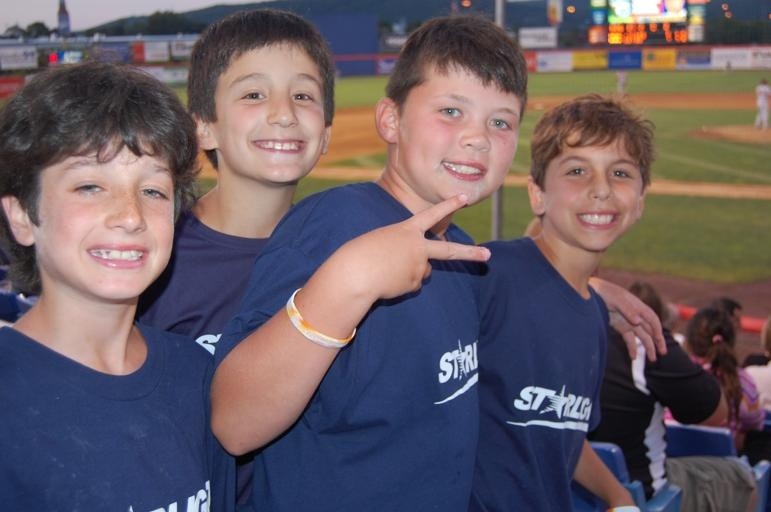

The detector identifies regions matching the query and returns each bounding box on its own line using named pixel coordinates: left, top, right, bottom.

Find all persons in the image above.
left=0, top=6, right=771, bottom=512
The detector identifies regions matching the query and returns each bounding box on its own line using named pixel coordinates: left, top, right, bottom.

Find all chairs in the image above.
left=0, top=244, right=41, bottom=328
left=568, top=406, right=771, bottom=512
